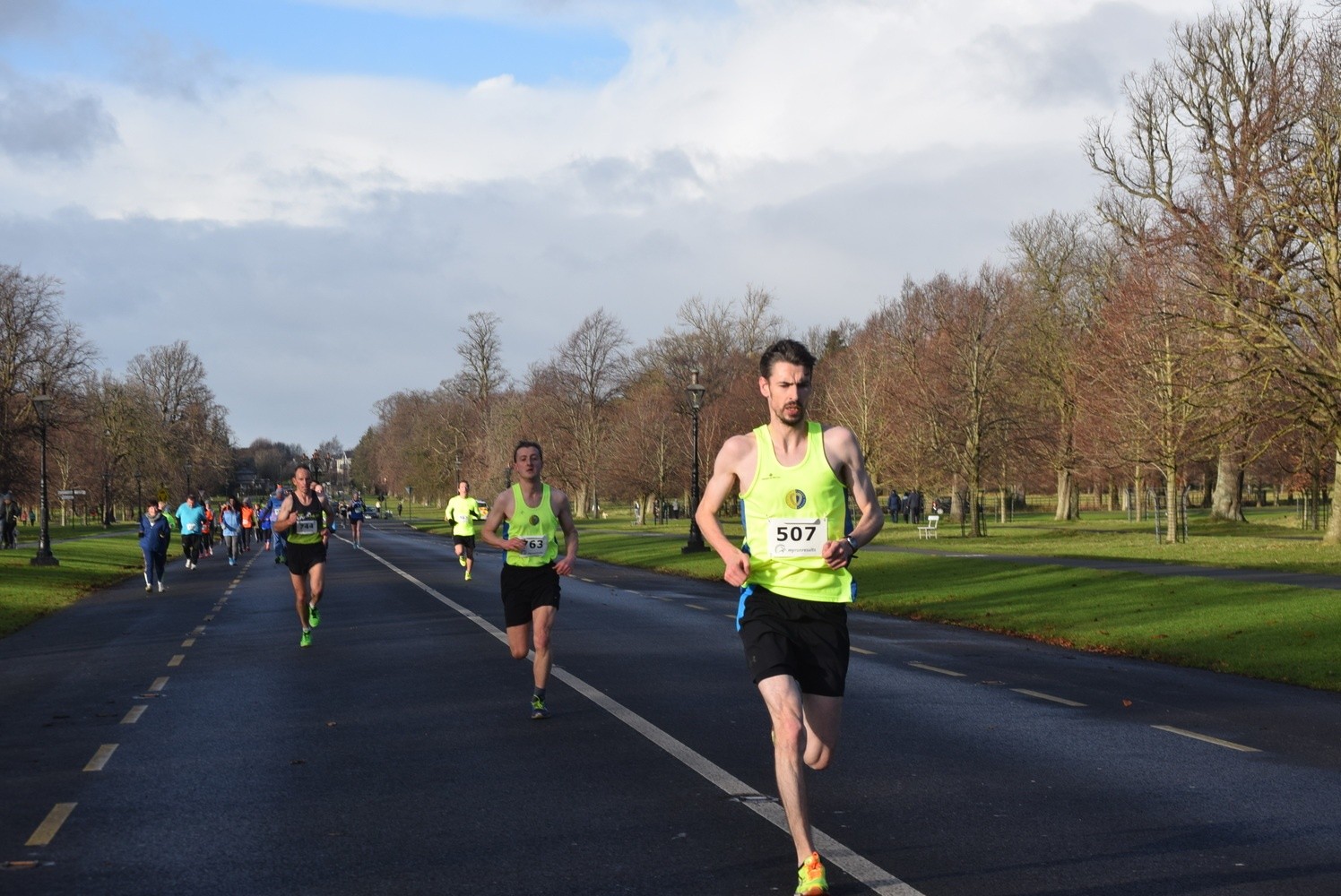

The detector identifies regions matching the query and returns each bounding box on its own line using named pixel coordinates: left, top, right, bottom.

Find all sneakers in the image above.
left=530, top=694, right=551, bottom=718
left=191, top=563, right=196, bottom=571
left=459, top=553, right=466, bottom=567
left=300, top=627, right=312, bottom=647
left=209, top=546, right=214, bottom=556
left=185, top=559, right=191, bottom=568
left=205, top=550, right=209, bottom=557
left=465, top=571, right=472, bottom=581
left=199, top=553, right=203, bottom=559
left=229, top=559, right=237, bottom=566
left=308, top=602, right=321, bottom=628
left=794, top=852, right=830, bottom=896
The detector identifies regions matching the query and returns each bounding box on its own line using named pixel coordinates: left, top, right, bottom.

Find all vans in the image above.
left=471, top=501, right=488, bottom=520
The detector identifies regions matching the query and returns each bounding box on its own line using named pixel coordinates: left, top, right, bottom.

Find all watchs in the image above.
left=843, top=536, right=859, bottom=559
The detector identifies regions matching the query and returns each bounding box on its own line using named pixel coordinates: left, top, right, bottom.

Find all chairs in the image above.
left=916, top=515, right=940, bottom=541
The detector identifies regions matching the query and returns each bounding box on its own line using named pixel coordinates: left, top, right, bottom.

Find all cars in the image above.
left=931, top=496, right=983, bottom=515
left=363, top=505, right=376, bottom=518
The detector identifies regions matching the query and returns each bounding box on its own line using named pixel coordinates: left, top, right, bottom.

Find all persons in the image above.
left=273, top=463, right=332, bottom=647
left=397, top=501, right=402, bottom=517
left=0, top=492, right=35, bottom=549
left=444, top=479, right=481, bottom=581
left=139, top=480, right=365, bottom=593
left=693, top=337, right=885, bottom=895
left=633, top=497, right=680, bottom=519
left=482, top=438, right=579, bottom=721
left=887, top=486, right=924, bottom=524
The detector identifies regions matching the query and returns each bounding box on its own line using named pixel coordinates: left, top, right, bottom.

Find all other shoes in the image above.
left=145, top=583, right=152, bottom=593
left=158, top=581, right=166, bottom=593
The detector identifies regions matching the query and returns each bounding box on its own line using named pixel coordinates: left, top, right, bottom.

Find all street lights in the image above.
left=134, top=470, right=143, bottom=522
left=681, top=365, right=711, bottom=554
left=30, top=379, right=60, bottom=565
